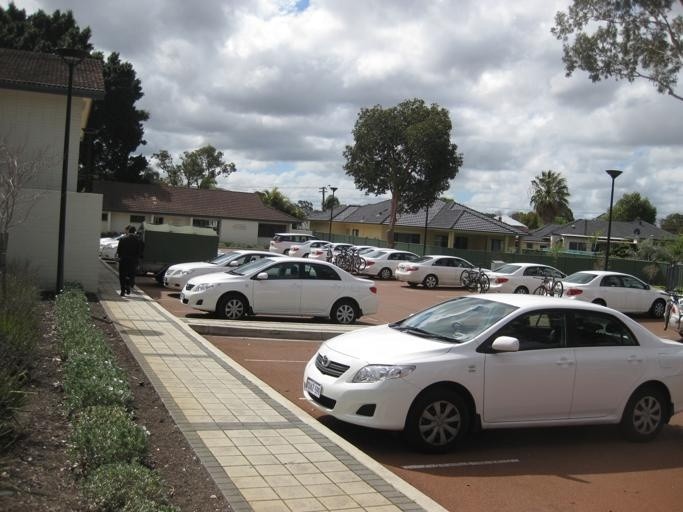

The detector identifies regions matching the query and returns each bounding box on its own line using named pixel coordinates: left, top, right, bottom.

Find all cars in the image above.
left=664, top=294, right=683, bottom=338
left=102, top=233, right=126, bottom=260
left=302, top=293, right=683, bottom=453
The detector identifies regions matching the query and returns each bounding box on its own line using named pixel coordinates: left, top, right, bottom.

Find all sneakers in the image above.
left=120, top=282, right=136, bottom=296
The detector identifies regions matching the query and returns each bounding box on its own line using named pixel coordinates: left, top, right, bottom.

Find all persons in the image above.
left=130, top=231, right=144, bottom=288
left=123, top=225, right=132, bottom=294
left=116, top=226, right=139, bottom=297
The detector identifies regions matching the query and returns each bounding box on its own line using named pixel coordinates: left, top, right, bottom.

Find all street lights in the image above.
left=328, top=187, right=337, bottom=241
left=604, top=170, right=622, bottom=271
